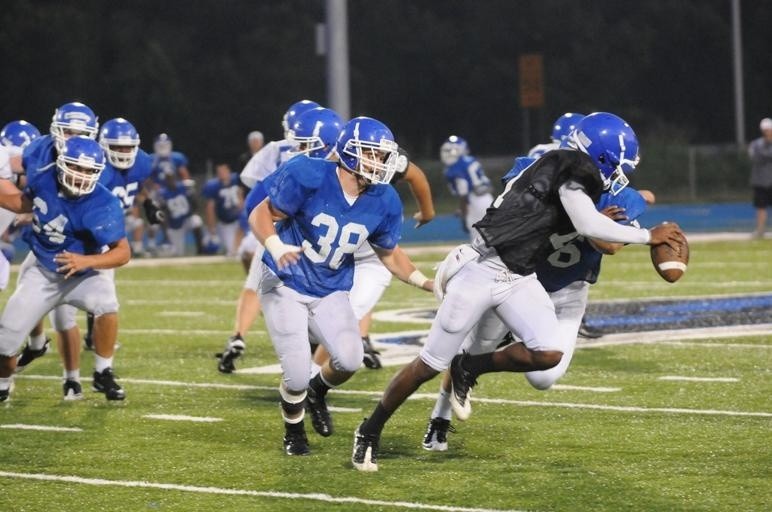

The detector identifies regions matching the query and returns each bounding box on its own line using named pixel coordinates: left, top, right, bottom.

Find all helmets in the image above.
left=0, top=120, right=41, bottom=148
left=335, top=116, right=399, bottom=185
left=49, top=102, right=99, bottom=155
left=98, top=117, right=140, bottom=169
left=551, top=113, right=586, bottom=148
left=440, top=135, right=467, bottom=164
left=286, top=107, right=343, bottom=160
left=281, top=99, right=321, bottom=139
left=56, top=135, right=106, bottom=197
left=563, top=111, right=640, bottom=196
left=150, top=131, right=176, bottom=183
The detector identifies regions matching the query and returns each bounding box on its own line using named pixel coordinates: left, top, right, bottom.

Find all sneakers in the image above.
left=421, top=416, right=458, bottom=452
left=361, top=336, right=382, bottom=370
left=130, top=233, right=239, bottom=261
left=304, top=381, right=333, bottom=438
left=352, top=417, right=381, bottom=473
left=448, top=349, right=480, bottom=422
left=283, top=421, right=310, bottom=457
left=1, top=336, right=125, bottom=403
left=218, top=336, right=245, bottom=374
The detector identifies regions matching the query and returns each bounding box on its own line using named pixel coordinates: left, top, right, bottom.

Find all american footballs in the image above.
left=650, top=221, right=688, bottom=283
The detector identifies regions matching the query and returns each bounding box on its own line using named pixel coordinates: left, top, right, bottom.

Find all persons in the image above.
left=746, top=118, right=772, bottom=240
left=1, top=97, right=267, bottom=405
left=213, top=99, right=687, bottom=473
